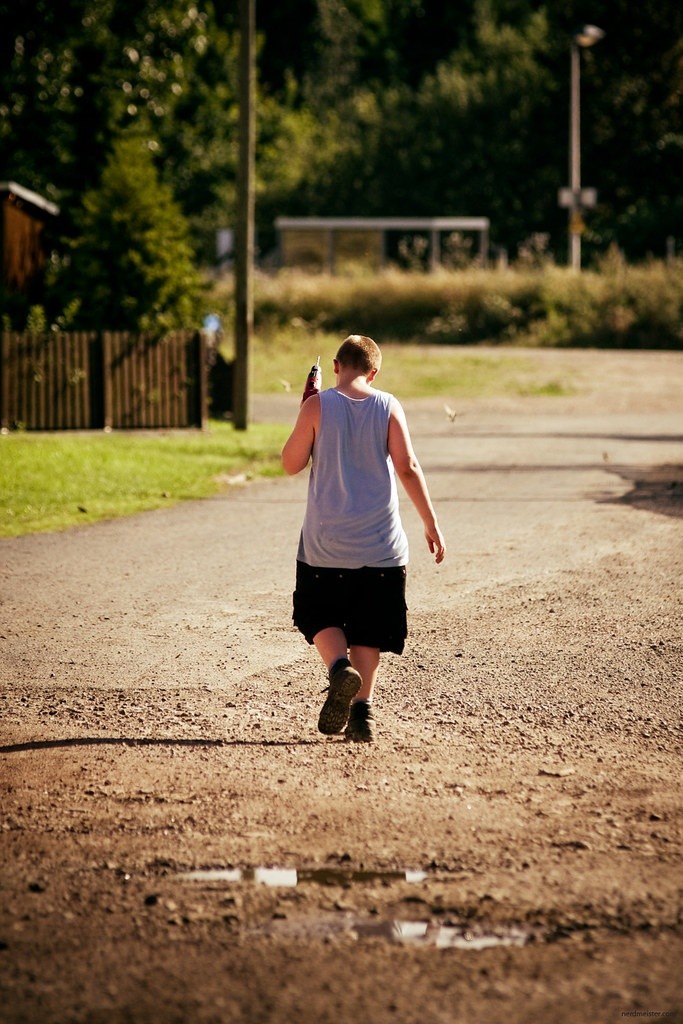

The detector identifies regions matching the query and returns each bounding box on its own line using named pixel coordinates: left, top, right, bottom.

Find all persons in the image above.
left=280, top=335, right=446, bottom=743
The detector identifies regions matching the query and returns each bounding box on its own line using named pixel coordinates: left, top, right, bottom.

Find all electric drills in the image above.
left=302, top=354, right=323, bottom=402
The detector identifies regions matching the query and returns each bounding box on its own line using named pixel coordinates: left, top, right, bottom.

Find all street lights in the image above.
left=571, top=23, right=604, bottom=275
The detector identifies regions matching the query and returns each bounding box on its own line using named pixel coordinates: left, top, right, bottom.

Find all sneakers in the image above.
left=318, top=658, right=363, bottom=736
left=344, top=702, right=377, bottom=742
